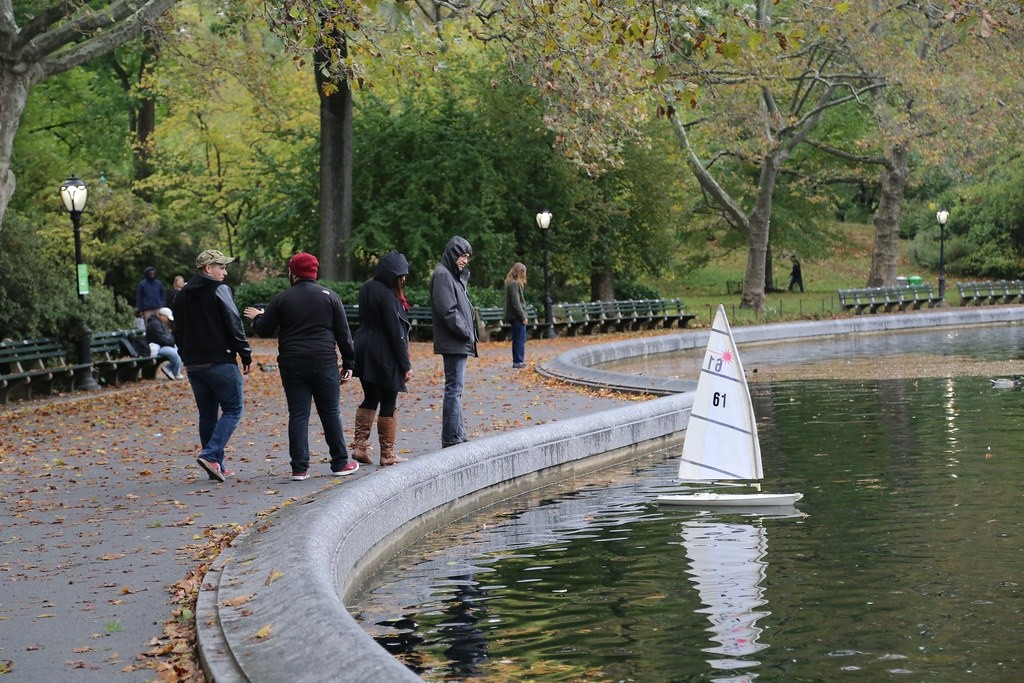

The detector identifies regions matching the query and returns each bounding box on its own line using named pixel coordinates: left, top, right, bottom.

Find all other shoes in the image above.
left=291, top=469, right=311, bottom=480
left=207, top=469, right=235, bottom=479
left=160, top=365, right=175, bottom=380
left=333, top=459, right=360, bottom=477
left=175, top=373, right=184, bottom=380
left=197, top=456, right=226, bottom=482
left=512, top=363, right=528, bottom=369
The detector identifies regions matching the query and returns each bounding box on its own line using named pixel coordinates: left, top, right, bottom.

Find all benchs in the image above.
left=835, top=280, right=1024, bottom=313
left=249, top=291, right=700, bottom=341
left=0, top=326, right=164, bottom=406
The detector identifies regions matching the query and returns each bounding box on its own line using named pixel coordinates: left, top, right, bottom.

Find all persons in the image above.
left=146, top=307, right=184, bottom=380
left=504, top=262, right=528, bottom=369
left=787, top=256, right=803, bottom=292
left=243, top=254, right=359, bottom=480
left=137, top=267, right=166, bottom=323
left=429, top=236, right=479, bottom=449
left=172, top=250, right=252, bottom=482
left=351, top=252, right=409, bottom=466
left=167, top=276, right=185, bottom=307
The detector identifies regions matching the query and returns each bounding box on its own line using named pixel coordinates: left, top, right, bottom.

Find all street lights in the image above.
left=535, top=206, right=557, bottom=338
left=935, top=204, right=951, bottom=309
left=59, top=173, right=101, bottom=389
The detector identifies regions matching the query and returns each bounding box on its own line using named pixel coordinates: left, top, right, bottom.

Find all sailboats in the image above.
left=657, top=304, right=803, bottom=507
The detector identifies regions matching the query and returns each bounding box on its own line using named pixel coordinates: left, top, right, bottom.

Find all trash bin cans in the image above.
left=896, top=275, right=922, bottom=284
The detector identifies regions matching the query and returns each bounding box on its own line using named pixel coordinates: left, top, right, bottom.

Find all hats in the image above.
left=195, top=249, right=235, bottom=268
left=160, top=308, right=175, bottom=322
left=288, top=252, right=319, bottom=279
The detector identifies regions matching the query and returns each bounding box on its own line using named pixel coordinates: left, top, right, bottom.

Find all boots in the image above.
left=351, top=408, right=375, bottom=464
left=377, top=406, right=409, bottom=466
